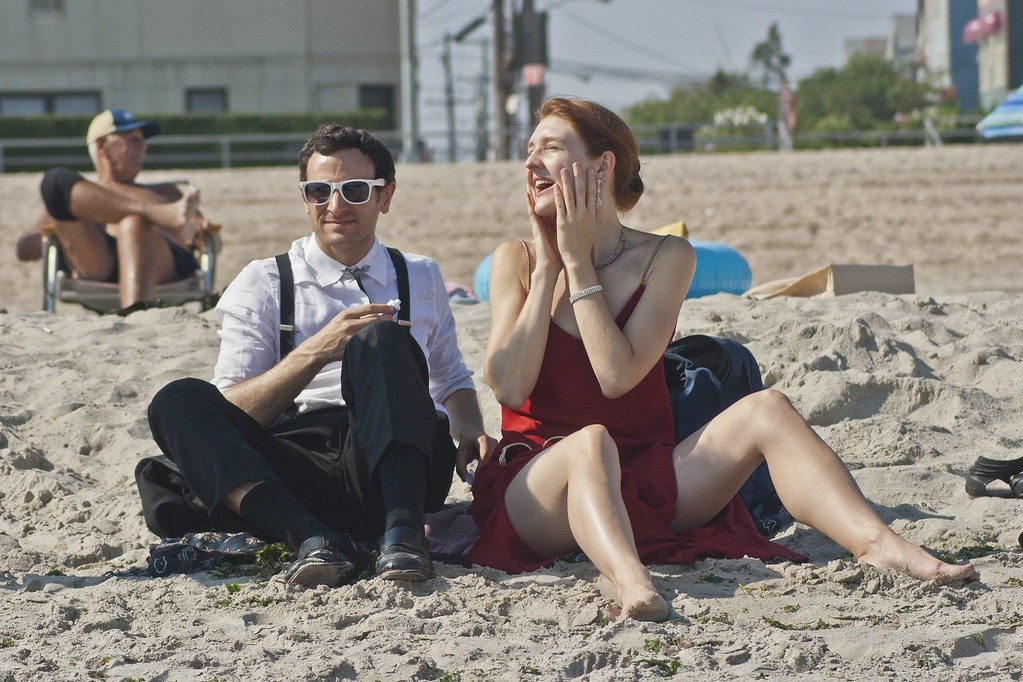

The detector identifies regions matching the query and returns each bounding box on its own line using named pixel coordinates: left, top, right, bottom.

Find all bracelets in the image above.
left=569, top=285, right=602, bottom=304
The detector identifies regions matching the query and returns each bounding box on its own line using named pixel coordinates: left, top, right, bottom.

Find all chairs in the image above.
left=42, top=182, right=216, bottom=313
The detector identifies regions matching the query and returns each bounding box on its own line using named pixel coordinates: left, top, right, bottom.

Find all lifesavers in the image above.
left=473, top=239, right=753, bottom=305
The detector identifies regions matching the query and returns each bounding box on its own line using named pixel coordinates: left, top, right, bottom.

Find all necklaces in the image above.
left=594, top=229, right=625, bottom=270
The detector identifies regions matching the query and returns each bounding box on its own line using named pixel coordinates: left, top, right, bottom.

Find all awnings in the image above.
left=965, top=13, right=1000, bottom=43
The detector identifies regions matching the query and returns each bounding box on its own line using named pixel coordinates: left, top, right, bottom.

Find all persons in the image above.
left=148, top=123, right=500, bottom=582
left=463, top=97, right=975, bottom=622
left=15, top=110, right=221, bottom=312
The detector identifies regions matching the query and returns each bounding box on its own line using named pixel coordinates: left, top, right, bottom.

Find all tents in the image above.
left=975, top=85, right=1023, bottom=140
